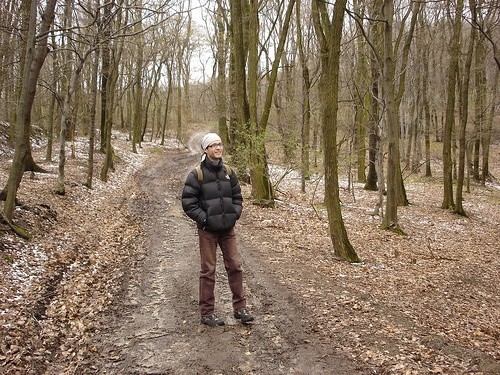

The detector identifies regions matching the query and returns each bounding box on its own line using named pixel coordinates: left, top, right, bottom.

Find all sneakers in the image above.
left=234, top=308, right=254, bottom=324
left=201, top=313, right=223, bottom=326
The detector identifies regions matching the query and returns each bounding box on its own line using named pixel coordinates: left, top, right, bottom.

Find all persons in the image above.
left=181, top=132, right=254, bottom=327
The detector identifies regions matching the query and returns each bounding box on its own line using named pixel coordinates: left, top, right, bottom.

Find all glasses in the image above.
left=207, top=143, right=223, bottom=149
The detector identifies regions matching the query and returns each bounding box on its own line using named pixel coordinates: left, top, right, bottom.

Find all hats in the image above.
left=201, top=133, right=221, bottom=150
